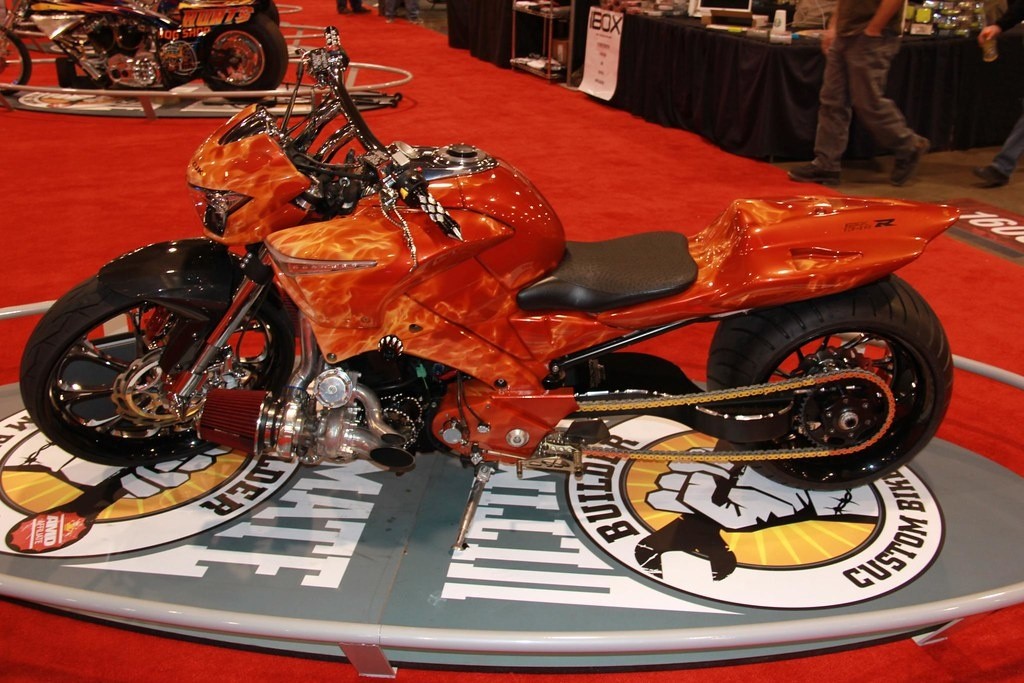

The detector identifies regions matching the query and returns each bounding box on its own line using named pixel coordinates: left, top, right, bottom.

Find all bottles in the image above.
left=599, top=0, right=687, bottom=17
left=982, top=33, right=998, bottom=62
left=904, top=0, right=987, bottom=38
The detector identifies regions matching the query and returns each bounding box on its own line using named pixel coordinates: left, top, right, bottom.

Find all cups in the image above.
left=773, top=9, right=787, bottom=35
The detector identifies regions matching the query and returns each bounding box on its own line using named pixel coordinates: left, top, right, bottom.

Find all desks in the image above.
left=446, top=0, right=601, bottom=68
left=605, top=11, right=1023, bottom=165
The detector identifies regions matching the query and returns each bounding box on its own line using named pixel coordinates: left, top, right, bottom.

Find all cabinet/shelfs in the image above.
left=510, top=0, right=575, bottom=87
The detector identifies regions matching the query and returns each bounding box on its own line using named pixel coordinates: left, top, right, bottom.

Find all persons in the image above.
left=337, top=0, right=372, bottom=14
left=968, top=0, right=1024, bottom=184
left=378, top=0, right=420, bottom=18
left=786, top=0, right=929, bottom=183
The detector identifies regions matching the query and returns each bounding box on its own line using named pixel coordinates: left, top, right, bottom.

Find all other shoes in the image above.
left=788, top=164, right=839, bottom=184
left=890, top=138, right=929, bottom=184
left=974, top=167, right=1008, bottom=187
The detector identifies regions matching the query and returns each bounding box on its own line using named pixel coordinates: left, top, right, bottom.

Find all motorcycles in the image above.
left=15, top=24, right=962, bottom=493
left=0, top=0, right=291, bottom=105
